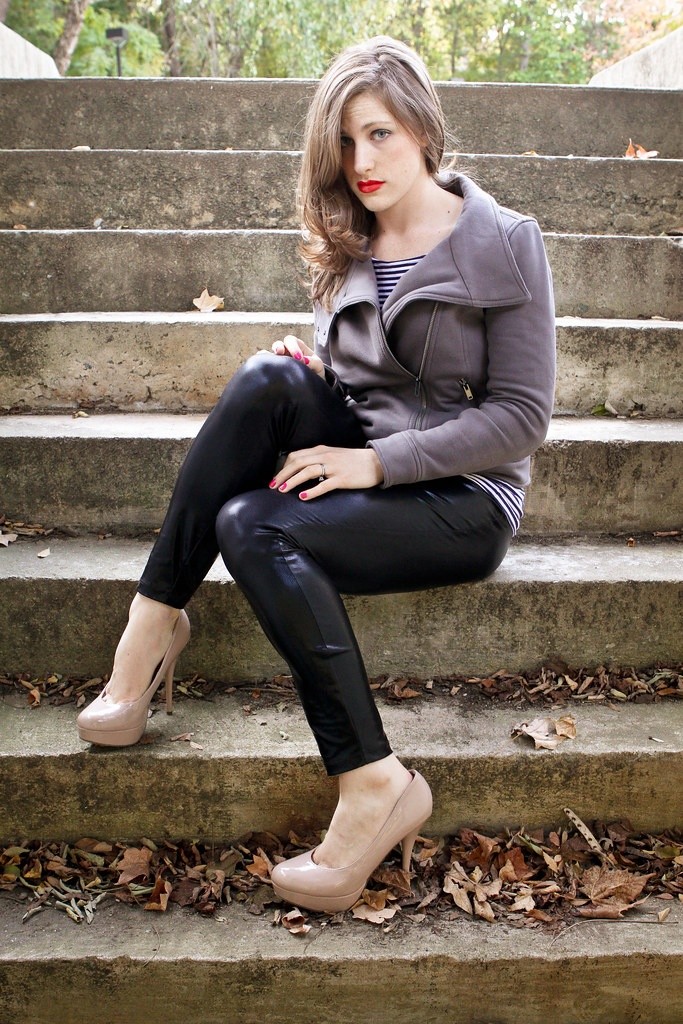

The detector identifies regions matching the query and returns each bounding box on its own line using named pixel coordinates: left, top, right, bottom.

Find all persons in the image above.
left=76, top=37, right=558, bottom=912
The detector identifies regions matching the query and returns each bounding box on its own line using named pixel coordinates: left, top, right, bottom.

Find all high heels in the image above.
left=77, top=609, right=191, bottom=747
left=272, top=767, right=432, bottom=913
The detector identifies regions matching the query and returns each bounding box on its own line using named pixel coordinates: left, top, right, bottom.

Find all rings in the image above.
left=319, top=464, right=326, bottom=481
